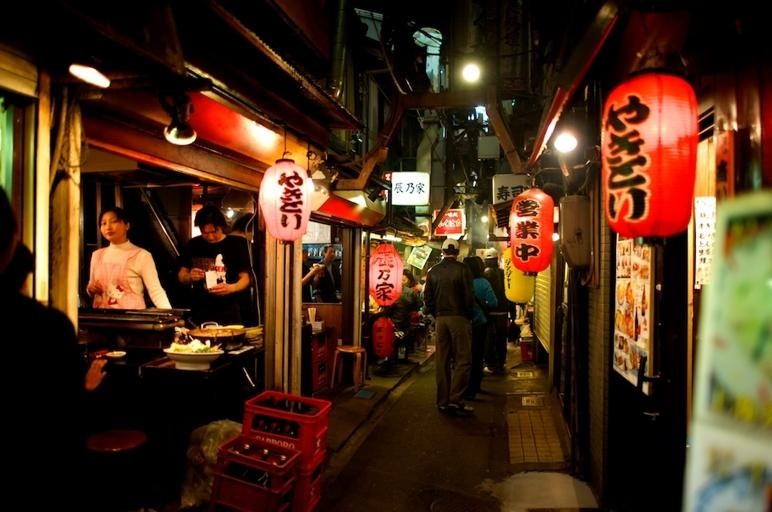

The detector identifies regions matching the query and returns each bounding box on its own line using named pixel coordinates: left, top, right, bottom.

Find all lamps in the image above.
left=159, top=93, right=199, bottom=146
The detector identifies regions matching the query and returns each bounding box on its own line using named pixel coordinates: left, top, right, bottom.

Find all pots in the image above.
left=186, top=321, right=247, bottom=344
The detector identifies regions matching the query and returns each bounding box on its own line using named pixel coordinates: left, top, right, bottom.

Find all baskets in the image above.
left=213, top=389, right=331, bottom=511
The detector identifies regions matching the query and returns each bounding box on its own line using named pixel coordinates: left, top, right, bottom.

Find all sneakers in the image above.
left=447, top=403, right=473, bottom=412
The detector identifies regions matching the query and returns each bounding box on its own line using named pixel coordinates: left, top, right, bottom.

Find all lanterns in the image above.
left=596, top=67, right=699, bottom=253
left=502, top=247, right=535, bottom=308
left=258, top=156, right=316, bottom=246
left=507, top=186, right=555, bottom=280
left=373, top=316, right=395, bottom=360
left=368, top=239, right=404, bottom=310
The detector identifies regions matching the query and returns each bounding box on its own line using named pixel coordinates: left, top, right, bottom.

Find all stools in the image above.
left=330, top=346, right=367, bottom=393
left=84, top=428, right=149, bottom=512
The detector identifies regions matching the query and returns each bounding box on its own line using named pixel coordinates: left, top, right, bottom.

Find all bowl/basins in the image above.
left=162, top=348, right=225, bottom=371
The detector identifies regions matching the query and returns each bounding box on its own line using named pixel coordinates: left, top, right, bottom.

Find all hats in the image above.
left=484, top=248, right=497, bottom=259
left=442, top=238, right=459, bottom=250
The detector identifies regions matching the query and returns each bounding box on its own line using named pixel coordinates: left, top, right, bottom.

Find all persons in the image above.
left=402, top=268, right=431, bottom=348
left=483, top=245, right=518, bottom=376
left=318, top=246, right=343, bottom=303
left=230, top=212, right=254, bottom=261
left=462, top=252, right=500, bottom=402
left=383, top=286, right=424, bottom=355
left=302, top=248, right=324, bottom=303
left=424, top=238, right=475, bottom=414
left=0, top=185, right=116, bottom=512
left=175, top=201, right=259, bottom=326
left=86, top=205, right=192, bottom=341
